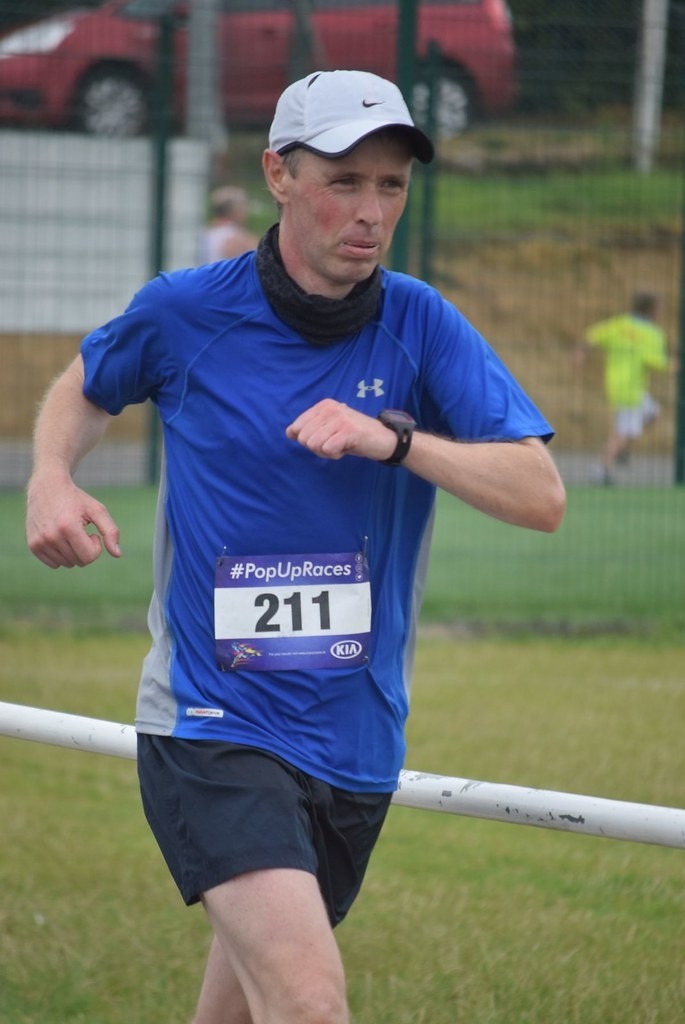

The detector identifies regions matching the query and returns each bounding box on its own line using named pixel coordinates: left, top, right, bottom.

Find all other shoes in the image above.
left=599, top=472, right=624, bottom=486
left=616, top=453, right=630, bottom=473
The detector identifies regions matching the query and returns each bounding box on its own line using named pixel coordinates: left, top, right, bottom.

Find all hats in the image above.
left=268, top=69, right=435, bottom=165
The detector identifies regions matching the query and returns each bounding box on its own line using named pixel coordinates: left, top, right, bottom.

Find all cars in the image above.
left=0, top=1, right=520, bottom=140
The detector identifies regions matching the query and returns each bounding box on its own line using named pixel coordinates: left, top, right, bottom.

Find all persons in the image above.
left=195, top=187, right=260, bottom=266
left=583, top=292, right=679, bottom=487
left=25, top=70, right=568, bottom=1024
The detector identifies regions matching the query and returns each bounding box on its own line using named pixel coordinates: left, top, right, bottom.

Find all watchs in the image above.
left=376, top=408, right=417, bottom=467
